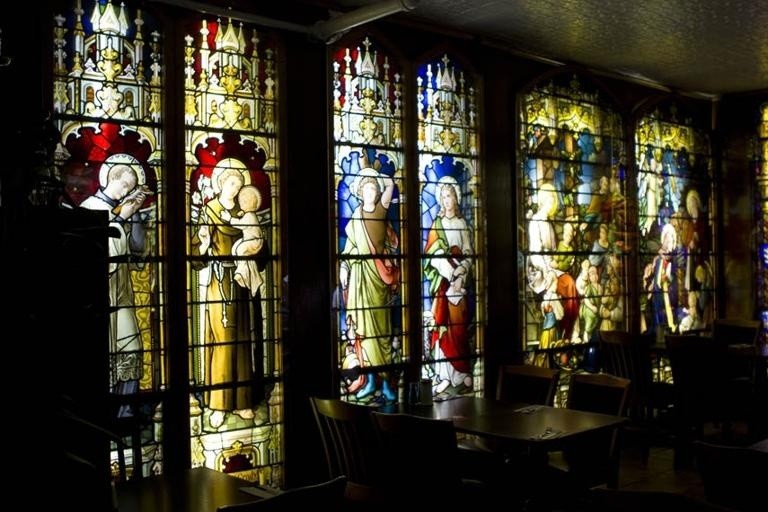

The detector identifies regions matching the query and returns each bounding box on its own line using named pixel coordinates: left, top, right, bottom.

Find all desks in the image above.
left=392, top=395, right=628, bottom=512
left=71, top=467, right=284, bottom=512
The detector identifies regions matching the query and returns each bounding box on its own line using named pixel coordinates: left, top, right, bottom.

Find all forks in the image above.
left=541, top=425, right=552, bottom=438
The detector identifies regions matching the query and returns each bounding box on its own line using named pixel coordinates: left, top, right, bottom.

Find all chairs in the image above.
left=310, top=396, right=501, bottom=511
left=699, top=442, right=768, bottom=512
left=496, top=363, right=631, bottom=491
left=593, top=316, right=764, bottom=464
left=218, top=476, right=347, bottom=512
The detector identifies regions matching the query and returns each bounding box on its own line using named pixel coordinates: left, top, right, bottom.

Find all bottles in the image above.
left=407, top=382, right=418, bottom=404
left=417, top=378, right=433, bottom=406
